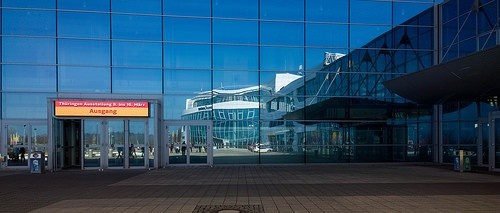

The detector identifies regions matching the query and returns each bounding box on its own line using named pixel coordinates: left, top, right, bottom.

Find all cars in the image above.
left=253, top=144, right=272, bottom=153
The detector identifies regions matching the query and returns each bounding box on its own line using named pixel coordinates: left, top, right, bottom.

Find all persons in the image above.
left=2, top=135, right=210, bottom=176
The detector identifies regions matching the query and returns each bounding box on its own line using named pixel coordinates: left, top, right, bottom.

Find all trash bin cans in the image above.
left=454, top=149, right=473, bottom=172
left=30, top=150, right=45, bottom=174
